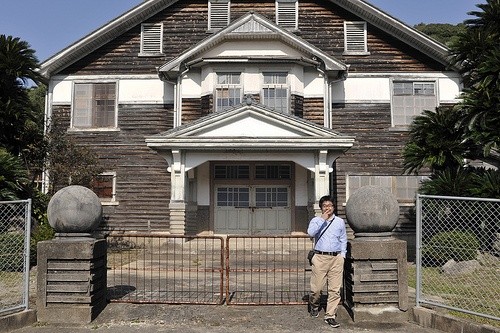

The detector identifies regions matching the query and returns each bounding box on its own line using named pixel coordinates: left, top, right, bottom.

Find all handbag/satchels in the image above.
left=307, top=251, right=315, bottom=266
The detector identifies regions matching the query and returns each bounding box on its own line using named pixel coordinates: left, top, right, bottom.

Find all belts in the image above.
left=315, top=250, right=342, bottom=256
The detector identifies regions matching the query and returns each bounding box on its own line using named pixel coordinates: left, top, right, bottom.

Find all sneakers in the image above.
left=324, top=318, right=341, bottom=328
left=311, top=307, right=319, bottom=318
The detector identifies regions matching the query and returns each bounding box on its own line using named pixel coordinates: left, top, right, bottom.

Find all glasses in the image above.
left=321, top=204, right=334, bottom=208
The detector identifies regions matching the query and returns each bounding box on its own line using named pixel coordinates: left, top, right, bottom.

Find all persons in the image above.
left=307, top=195, right=348, bottom=328
